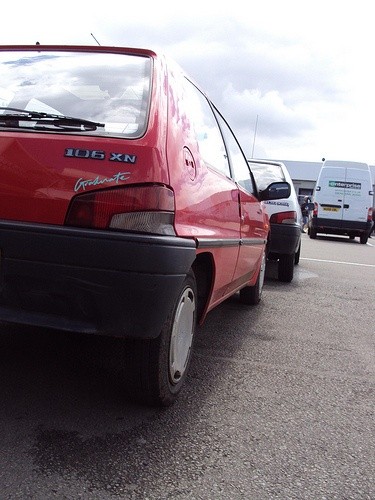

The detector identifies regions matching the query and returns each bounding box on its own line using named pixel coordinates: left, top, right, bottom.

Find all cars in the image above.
left=1, top=43, right=291, bottom=404
left=245, top=158, right=315, bottom=282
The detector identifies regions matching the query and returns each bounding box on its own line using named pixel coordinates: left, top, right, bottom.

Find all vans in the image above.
left=308, top=160, right=374, bottom=243
